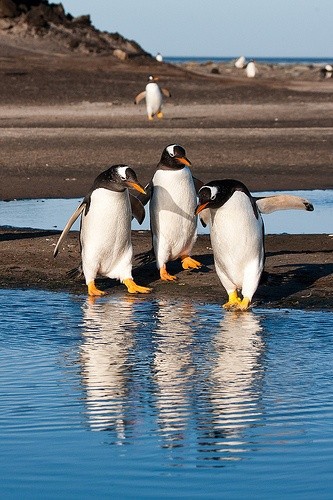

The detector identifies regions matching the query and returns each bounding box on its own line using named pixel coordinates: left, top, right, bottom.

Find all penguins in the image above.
left=138, top=143, right=204, bottom=283
left=53, top=164, right=154, bottom=296
left=155, top=53, right=163, bottom=62
left=133, top=74, right=172, bottom=122
left=243, top=59, right=259, bottom=79
left=193, top=178, right=314, bottom=313
left=234, top=56, right=247, bottom=69
